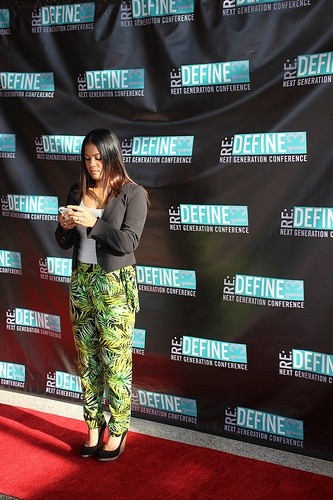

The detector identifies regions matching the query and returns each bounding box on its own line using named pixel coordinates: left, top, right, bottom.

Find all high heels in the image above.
left=97, top=429, right=128, bottom=461
left=80, top=420, right=106, bottom=458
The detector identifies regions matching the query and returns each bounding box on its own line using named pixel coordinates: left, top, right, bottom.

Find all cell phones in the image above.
left=59, top=208, right=75, bottom=220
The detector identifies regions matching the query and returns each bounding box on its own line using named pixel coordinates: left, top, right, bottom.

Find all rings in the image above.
left=63, top=223, right=66, bottom=226
left=77, top=218, right=82, bottom=221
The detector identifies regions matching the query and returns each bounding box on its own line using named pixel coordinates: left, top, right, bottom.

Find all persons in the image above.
left=55, top=127, right=150, bottom=463
